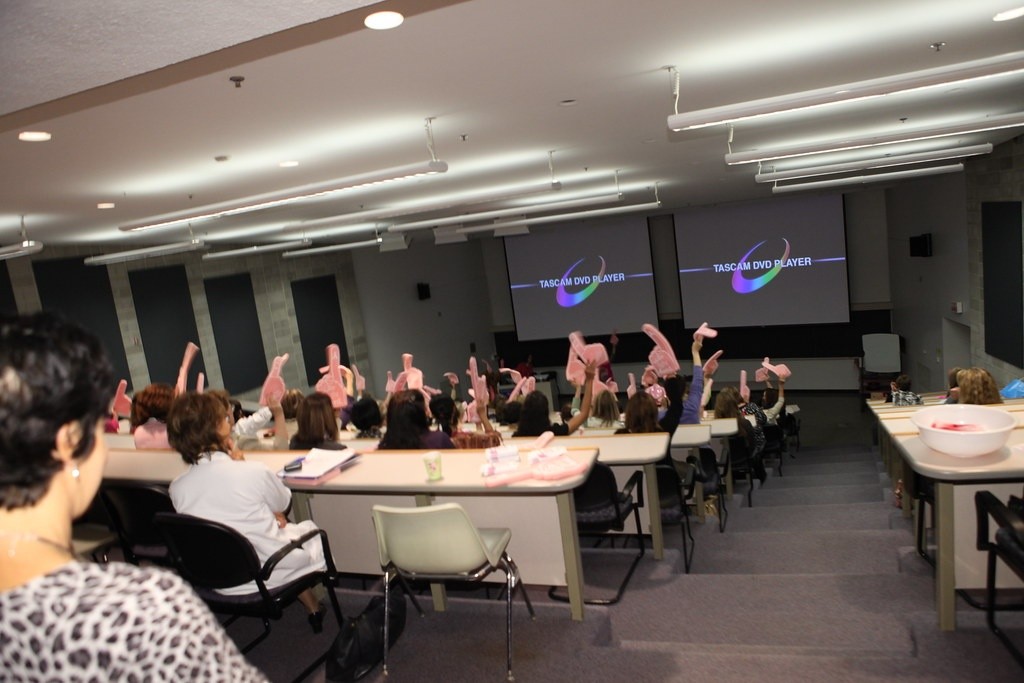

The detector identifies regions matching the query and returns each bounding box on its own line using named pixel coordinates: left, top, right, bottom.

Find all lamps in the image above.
left=0, top=116, right=663, bottom=265
left=665, top=51, right=1024, bottom=193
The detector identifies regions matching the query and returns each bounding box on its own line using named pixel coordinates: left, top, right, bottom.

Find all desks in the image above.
left=105, top=434, right=671, bottom=561
left=867, top=392, right=1024, bottom=631
left=708, top=405, right=801, bottom=425
left=703, top=418, right=741, bottom=502
left=461, top=422, right=712, bottom=525
left=101, top=449, right=600, bottom=621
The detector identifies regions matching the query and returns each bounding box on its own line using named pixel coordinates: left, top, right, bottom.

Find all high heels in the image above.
left=307, top=602, right=326, bottom=634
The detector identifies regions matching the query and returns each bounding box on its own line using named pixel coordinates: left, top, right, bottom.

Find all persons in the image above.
left=615, top=371, right=703, bottom=523
left=350, top=396, right=384, bottom=440
left=891, top=374, right=924, bottom=406
left=229, top=399, right=272, bottom=437
left=129, top=382, right=174, bottom=450
left=714, top=373, right=797, bottom=465
left=561, top=404, right=573, bottom=420
left=207, top=389, right=288, bottom=451
left=0, top=311, right=271, bottom=683
left=657, top=339, right=721, bottom=493
left=281, top=389, right=304, bottom=443
left=499, top=401, right=521, bottom=438
left=511, top=358, right=599, bottom=438
left=516, top=350, right=538, bottom=382
left=377, top=388, right=456, bottom=449
left=290, top=391, right=347, bottom=450
left=429, top=393, right=501, bottom=449
left=167, top=388, right=336, bottom=636
left=946, top=367, right=963, bottom=397
left=570, top=376, right=625, bottom=429
left=944, top=366, right=1005, bottom=404
left=700, top=377, right=713, bottom=409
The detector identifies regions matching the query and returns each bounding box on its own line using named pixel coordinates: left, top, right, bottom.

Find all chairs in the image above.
left=956, top=490, right=1024, bottom=669
left=857, top=334, right=907, bottom=411
left=913, top=470, right=936, bottom=568
left=71, top=413, right=802, bottom=683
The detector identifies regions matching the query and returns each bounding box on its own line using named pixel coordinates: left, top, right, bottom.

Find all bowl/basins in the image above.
left=910, top=403, right=1018, bottom=459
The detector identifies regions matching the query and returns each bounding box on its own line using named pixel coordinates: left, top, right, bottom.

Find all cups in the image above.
left=424, top=452, right=443, bottom=480
